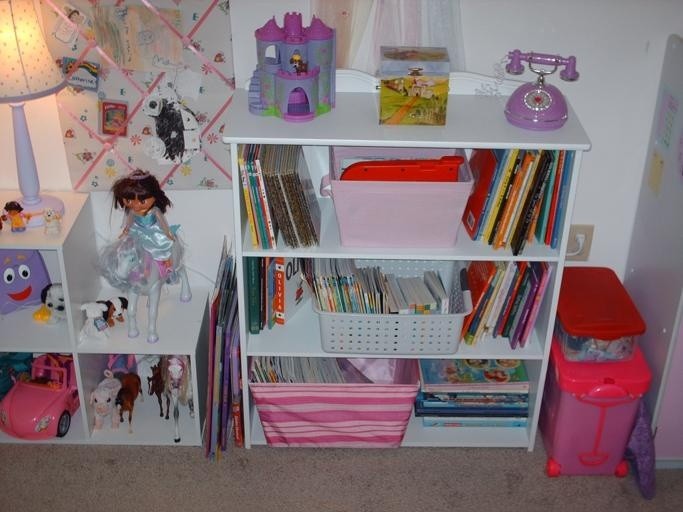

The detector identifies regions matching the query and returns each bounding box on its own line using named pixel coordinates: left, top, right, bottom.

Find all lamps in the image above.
left=1, top=1, right=70, bottom=226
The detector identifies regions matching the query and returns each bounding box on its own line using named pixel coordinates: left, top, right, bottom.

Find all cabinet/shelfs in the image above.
left=0, top=190, right=217, bottom=448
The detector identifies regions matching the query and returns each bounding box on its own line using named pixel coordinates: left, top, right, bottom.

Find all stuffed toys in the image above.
left=40, top=283, right=68, bottom=326
left=43, top=207, right=61, bottom=235
left=81, top=297, right=129, bottom=342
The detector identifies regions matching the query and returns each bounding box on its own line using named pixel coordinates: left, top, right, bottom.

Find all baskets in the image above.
left=309, top=257, right=474, bottom=356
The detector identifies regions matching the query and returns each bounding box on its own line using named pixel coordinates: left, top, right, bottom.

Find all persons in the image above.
left=0, top=200, right=32, bottom=233
left=94, top=170, right=192, bottom=344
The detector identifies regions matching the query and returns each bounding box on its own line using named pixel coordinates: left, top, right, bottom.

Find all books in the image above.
left=299, top=258, right=448, bottom=314
left=204, top=233, right=245, bottom=466
left=460, top=260, right=553, bottom=348
left=462, top=149, right=576, bottom=256
left=248, top=357, right=375, bottom=384
left=243, top=256, right=313, bottom=335
left=238, top=144, right=321, bottom=252
left=415, top=359, right=529, bottom=428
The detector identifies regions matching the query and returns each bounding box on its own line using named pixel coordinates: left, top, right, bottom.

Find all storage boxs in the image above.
left=223, top=70, right=592, bottom=450
left=318, top=146, right=477, bottom=253
left=542, top=264, right=652, bottom=480
